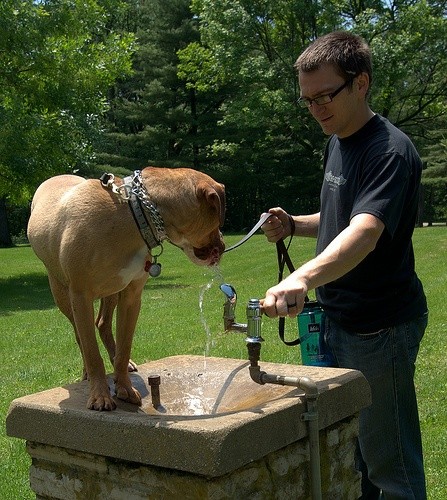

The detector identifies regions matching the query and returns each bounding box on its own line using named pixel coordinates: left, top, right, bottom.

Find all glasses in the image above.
left=296, top=81, right=349, bottom=109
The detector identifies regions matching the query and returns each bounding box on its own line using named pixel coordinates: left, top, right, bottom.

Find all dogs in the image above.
left=27, top=164, right=227, bottom=413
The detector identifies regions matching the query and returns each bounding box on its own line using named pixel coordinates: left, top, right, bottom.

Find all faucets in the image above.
left=215, top=282, right=250, bottom=332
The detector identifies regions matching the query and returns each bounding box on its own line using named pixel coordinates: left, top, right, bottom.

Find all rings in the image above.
left=287, top=302, right=296, bottom=309
left=257, top=30, right=430, bottom=500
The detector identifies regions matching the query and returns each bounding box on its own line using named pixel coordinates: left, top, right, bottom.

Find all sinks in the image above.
left=112, top=374, right=278, bottom=420
